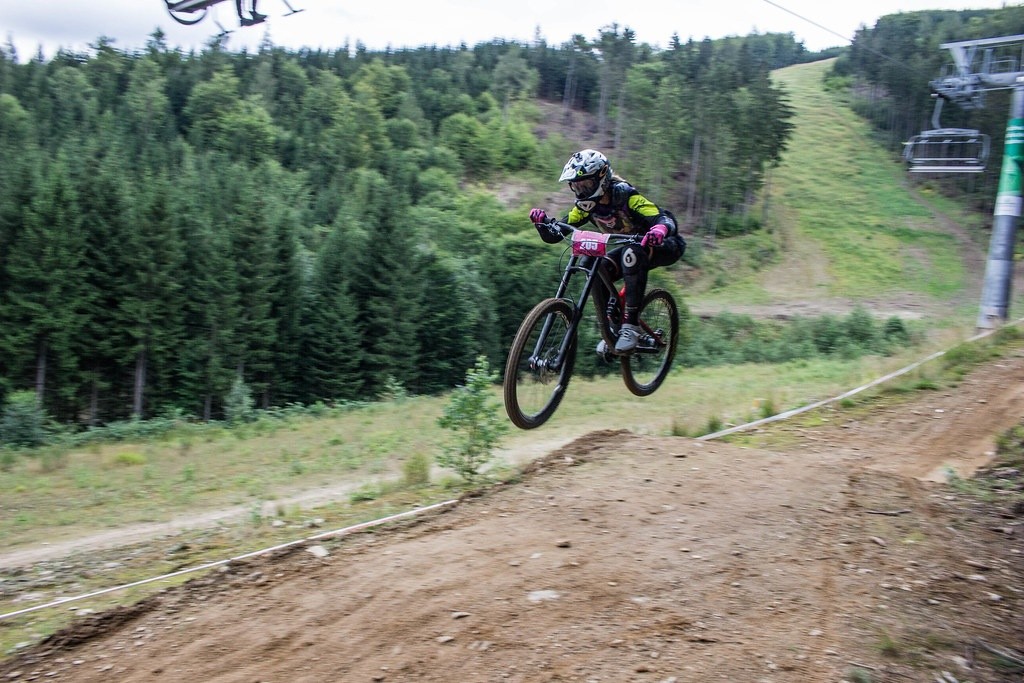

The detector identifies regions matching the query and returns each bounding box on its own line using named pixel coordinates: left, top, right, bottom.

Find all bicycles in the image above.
left=503, top=216, right=681, bottom=429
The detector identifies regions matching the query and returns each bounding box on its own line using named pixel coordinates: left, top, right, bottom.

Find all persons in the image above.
left=530, top=149, right=678, bottom=355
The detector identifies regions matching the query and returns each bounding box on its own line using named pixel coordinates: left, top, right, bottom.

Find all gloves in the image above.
left=530, top=208, right=548, bottom=228
left=641, top=224, right=668, bottom=248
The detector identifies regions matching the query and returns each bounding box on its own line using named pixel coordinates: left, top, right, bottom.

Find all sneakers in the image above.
left=596, top=327, right=618, bottom=355
left=614, top=323, right=643, bottom=353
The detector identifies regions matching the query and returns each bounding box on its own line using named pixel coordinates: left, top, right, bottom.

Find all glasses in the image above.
left=571, top=177, right=597, bottom=198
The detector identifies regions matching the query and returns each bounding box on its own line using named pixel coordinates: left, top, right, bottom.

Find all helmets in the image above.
left=559, top=149, right=612, bottom=212
